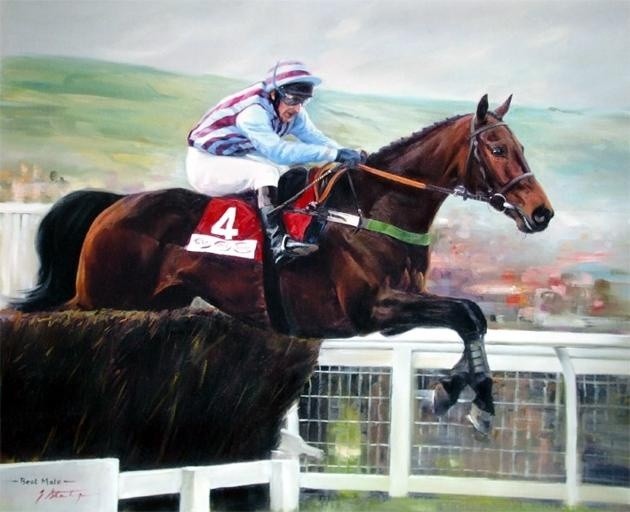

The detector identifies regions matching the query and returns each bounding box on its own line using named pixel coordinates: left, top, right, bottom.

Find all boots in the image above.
left=258, top=205, right=319, bottom=265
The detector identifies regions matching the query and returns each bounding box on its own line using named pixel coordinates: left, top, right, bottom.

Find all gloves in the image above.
left=334, top=149, right=368, bottom=167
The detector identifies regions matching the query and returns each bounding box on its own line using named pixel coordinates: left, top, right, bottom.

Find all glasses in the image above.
left=280, top=94, right=312, bottom=107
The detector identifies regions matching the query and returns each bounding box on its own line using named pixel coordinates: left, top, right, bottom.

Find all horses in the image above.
left=4, top=92, right=555, bottom=438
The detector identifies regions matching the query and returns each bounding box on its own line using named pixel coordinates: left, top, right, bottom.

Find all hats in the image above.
left=263, top=59, right=322, bottom=93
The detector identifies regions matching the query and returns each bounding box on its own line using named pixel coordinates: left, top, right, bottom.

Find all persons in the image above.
left=186, top=59, right=368, bottom=267
